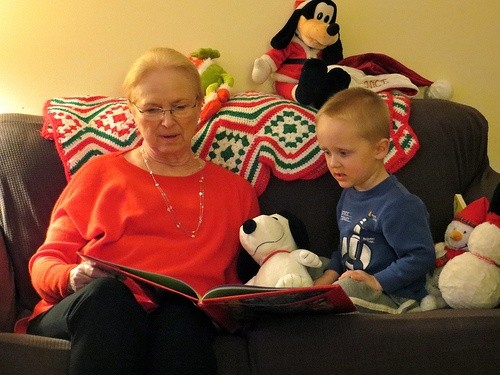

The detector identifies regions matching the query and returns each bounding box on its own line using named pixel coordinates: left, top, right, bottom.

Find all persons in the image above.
left=313, top=87, right=435, bottom=314
left=29, top=48, right=259, bottom=375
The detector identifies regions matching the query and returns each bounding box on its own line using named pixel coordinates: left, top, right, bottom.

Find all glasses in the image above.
left=132, top=96, right=200, bottom=121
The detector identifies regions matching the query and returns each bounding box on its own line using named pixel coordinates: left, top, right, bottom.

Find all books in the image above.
left=76, top=252, right=358, bottom=336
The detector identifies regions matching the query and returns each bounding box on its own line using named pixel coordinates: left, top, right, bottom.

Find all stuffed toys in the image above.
left=438, top=214, right=500, bottom=308
left=421, top=197, right=490, bottom=310
left=187, top=48, right=235, bottom=122
left=238, top=211, right=323, bottom=288
left=252, top=0, right=351, bottom=106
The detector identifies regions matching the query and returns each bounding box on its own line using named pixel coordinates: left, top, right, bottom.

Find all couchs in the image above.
left=0, top=99, right=500, bottom=375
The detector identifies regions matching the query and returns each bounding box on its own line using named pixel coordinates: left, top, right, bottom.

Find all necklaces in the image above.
left=140, top=147, right=205, bottom=238
